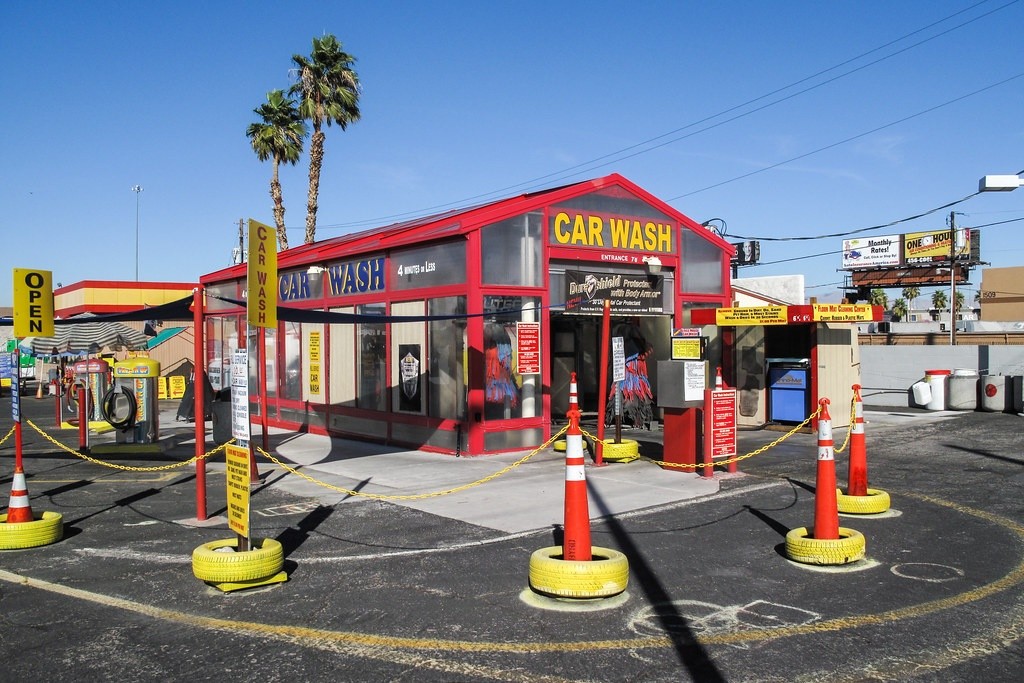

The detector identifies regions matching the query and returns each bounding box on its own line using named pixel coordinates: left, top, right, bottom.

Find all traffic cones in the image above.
left=6, top=466, right=34, bottom=523
left=34, top=383, right=45, bottom=399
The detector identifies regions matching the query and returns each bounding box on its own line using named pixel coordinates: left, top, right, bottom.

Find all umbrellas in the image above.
left=17, top=311, right=148, bottom=454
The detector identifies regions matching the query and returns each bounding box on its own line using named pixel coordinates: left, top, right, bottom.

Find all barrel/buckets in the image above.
left=1013, top=376, right=1024, bottom=415
left=933, top=368, right=980, bottom=411
left=925, top=369, right=950, bottom=412
left=982, top=374, right=1010, bottom=412
left=912, top=381, right=932, bottom=405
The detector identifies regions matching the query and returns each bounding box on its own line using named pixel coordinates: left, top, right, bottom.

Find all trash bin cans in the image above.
left=211, top=386, right=232, bottom=446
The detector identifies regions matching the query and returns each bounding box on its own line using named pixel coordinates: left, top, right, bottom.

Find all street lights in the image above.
left=132, top=185, right=145, bottom=282
